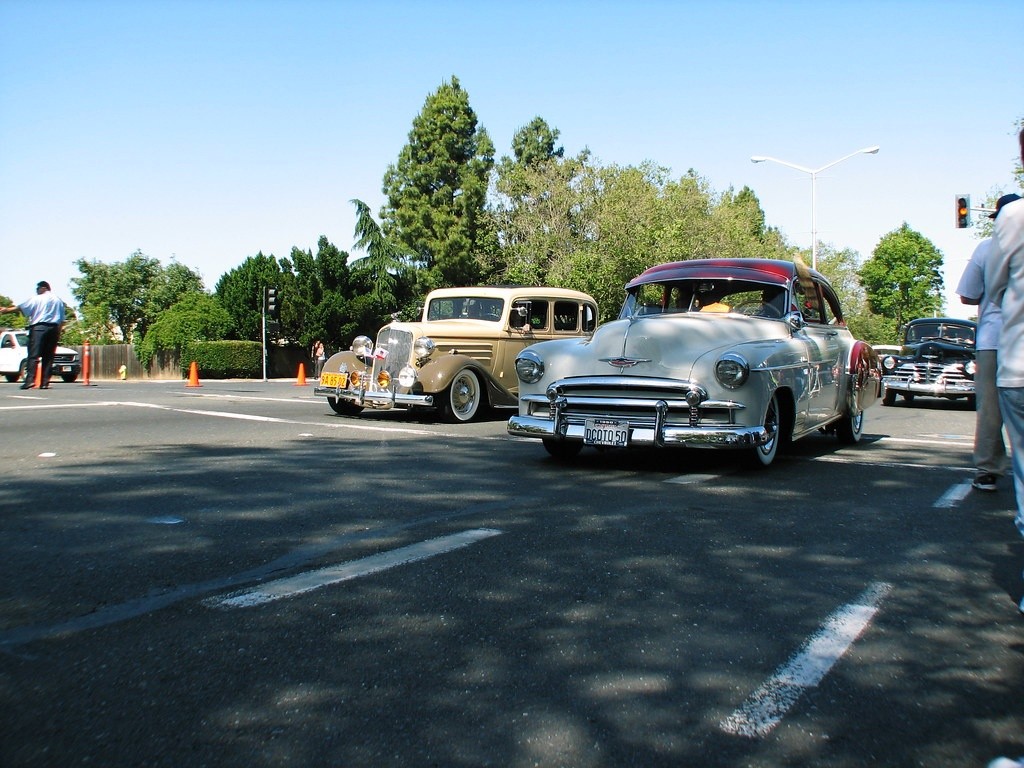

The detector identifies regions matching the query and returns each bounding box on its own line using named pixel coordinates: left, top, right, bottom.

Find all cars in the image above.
left=505, top=258, right=885, bottom=475
left=880, top=317, right=979, bottom=411
left=313, top=284, right=599, bottom=423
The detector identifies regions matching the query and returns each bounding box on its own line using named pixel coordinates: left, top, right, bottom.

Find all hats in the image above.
left=988, top=194, right=1022, bottom=218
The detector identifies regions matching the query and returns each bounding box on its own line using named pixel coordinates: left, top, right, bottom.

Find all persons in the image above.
left=311, top=339, right=325, bottom=379
left=0, top=281, right=64, bottom=390
left=761, top=288, right=800, bottom=319
left=955, top=129, right=1024, bottom=610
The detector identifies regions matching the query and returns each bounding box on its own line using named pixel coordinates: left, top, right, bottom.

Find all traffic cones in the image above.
left=292, top=362, right=311, bottom=387
left=33, top=364, right=42, bottom=389
left=185, top=361, right=204, bottom=387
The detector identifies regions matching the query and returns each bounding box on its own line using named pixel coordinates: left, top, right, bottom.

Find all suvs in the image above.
left=0, top=329, right=82, bottom=382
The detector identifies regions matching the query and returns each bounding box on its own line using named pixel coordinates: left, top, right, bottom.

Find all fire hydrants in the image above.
left=119, top=364, right=128, bottom=381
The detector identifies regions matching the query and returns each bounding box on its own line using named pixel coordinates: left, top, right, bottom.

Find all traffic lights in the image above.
left=263, top=287, right=278, bottom=313
left=955, top=193, right=970, bottom=227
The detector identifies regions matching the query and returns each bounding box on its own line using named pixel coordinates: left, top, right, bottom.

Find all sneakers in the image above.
left=971, top=472, right=997, bottom=490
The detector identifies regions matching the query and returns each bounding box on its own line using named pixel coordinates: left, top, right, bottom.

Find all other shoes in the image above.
left=19, top=382, right=35, bottom=390
left=40, top=385, right=47, bottom=389
left=1014, top=514, right=1024, bottom=536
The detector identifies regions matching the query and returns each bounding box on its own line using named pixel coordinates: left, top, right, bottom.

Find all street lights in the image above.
left=749, top=146, right=880, bottom=270
